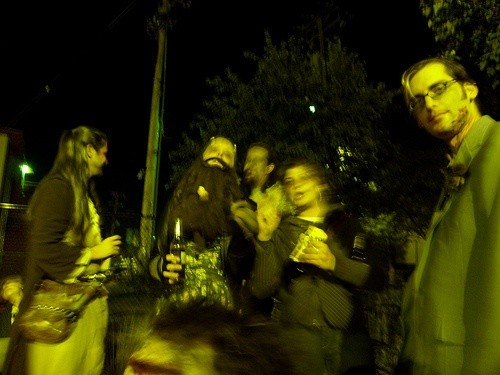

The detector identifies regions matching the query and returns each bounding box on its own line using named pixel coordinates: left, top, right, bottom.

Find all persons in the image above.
left=120, top=137, right=371, bottom=375
left=401, top=58, right=500, bottom=375
left=0, top=127, right=122, bottom=375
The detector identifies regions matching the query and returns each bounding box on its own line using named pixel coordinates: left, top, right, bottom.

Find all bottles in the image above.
left=170, top=217, right=186, bottom=278
left=100, top=223, right=116, bottom=272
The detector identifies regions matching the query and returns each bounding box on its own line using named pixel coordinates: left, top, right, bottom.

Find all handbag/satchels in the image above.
left=13, top=278, right=109, bottom=345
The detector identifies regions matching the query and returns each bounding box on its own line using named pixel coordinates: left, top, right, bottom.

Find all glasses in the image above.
left=408, top=77, right=457, bottom=112
